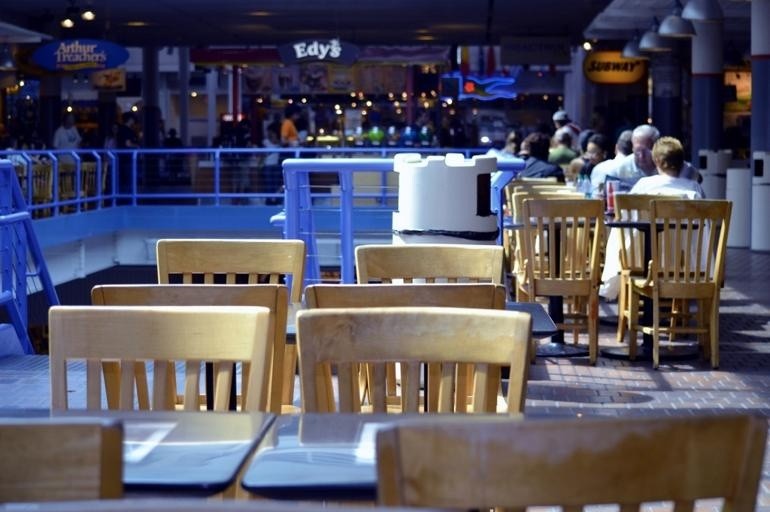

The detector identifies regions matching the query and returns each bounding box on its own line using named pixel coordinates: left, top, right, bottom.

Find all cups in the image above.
left=607, top=179, right=620, bottom=212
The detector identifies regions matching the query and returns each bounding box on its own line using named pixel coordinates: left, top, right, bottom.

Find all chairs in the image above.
left=376, top=418, right=769, bottom=512
left=14, top=161, right=109, bottom=220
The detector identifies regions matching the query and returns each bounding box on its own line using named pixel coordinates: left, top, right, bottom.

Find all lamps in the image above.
left=619, top=0, right=725, bottom=59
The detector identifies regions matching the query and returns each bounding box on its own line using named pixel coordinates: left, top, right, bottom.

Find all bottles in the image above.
left=583, top=175, right=592, bottom=200
left=577, top=174, right=584, bottom=193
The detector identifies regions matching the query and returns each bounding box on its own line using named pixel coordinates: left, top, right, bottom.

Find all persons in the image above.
left=53, top=96, right=302, bottom=205
left=626, top=137, right=705, bottom=351
left=496, top=110, right=643, bottom=347
left=605, top=125, right=700, bottom=193
left=396, top=106, right=453, bottom=148
